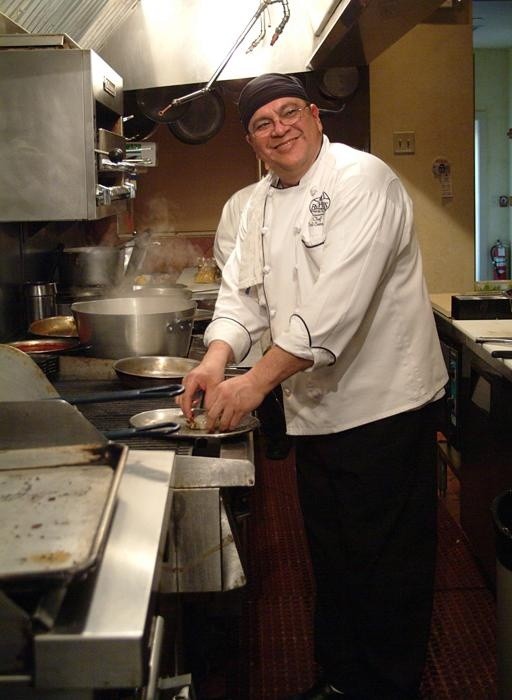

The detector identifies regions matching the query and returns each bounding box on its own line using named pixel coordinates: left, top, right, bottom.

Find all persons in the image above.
left=209, top=161, right=293, bottom=462
left=172, top=73, right=451, bottom=696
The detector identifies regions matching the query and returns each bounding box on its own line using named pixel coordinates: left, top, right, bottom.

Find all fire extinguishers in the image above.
left=490, top=239, right=507, bottom=280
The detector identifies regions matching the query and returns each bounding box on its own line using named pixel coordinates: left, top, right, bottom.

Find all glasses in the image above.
left=252, top=102, right=309, bottom=139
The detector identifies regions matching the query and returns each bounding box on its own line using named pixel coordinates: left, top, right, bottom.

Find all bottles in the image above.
left=25, top=280, right=58, bottom=322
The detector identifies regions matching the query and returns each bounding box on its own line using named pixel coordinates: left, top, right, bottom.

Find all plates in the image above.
left=129, top=406, right=260, bottom=439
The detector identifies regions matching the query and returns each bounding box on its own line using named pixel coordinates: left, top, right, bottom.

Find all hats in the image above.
left=235, top=72, right=311, bottom=137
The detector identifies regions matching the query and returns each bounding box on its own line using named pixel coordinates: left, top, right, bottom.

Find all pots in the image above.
left=112, top=355, right=254, bottom=386
left=71, top=295, right=199, bottom=358
left=123, top=85, right=229, bottom=147
left=61, top=227, right=155, bottom=287
